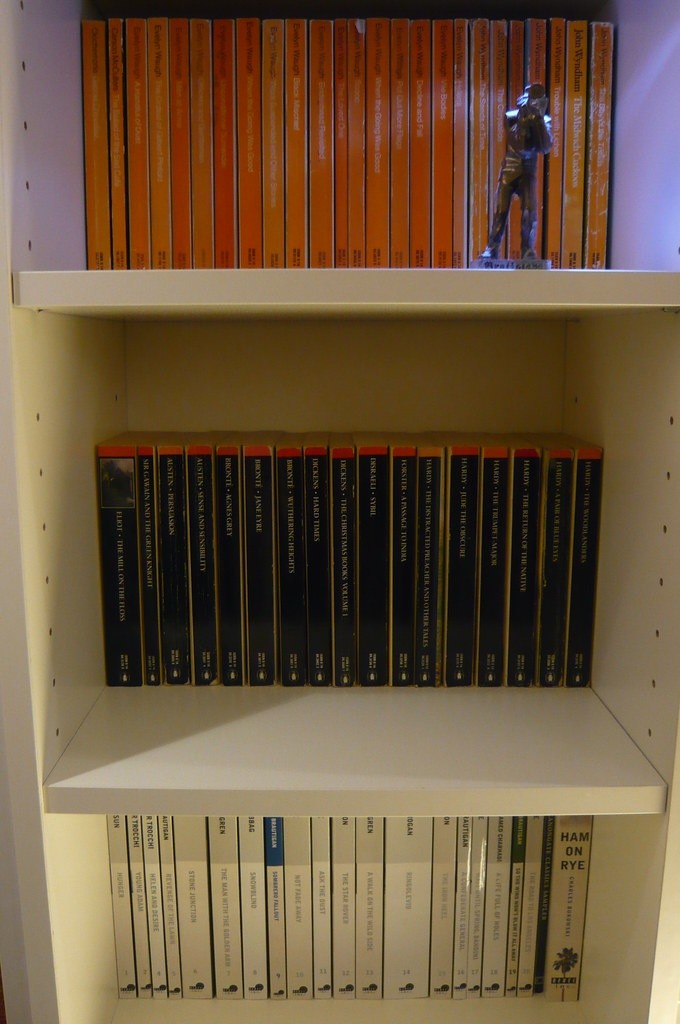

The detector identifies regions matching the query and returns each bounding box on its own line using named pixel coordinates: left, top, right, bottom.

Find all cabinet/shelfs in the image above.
left=0, top=0, right=680, bottom=1024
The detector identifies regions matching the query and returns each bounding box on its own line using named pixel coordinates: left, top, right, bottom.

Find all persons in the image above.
left=477, top=82, right=554, bottom=260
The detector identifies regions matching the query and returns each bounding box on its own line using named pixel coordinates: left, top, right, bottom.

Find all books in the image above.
left=106, top=815, right=592, bottom=1002
left=96, top=430, right=601, bottom=689
left=80, top=17, right=614, bottom=269
